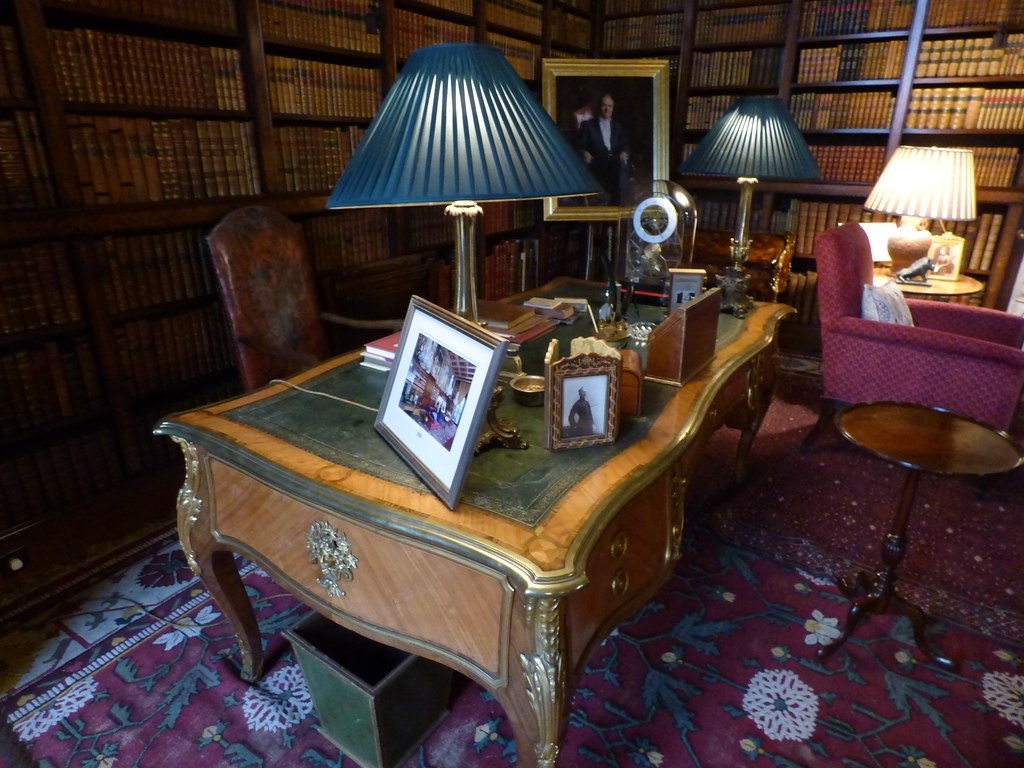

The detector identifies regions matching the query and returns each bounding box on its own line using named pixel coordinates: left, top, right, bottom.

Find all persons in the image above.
left=568, top=387, right=594, bottom=435
left=574, top=95, right=632, bottom=206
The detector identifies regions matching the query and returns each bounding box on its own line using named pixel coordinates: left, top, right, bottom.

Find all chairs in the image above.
left=682, top=228, right=796, bottom=303
left=802, top=223, right=1024, bottom=502
left=207, top=206, right=404, bottom=392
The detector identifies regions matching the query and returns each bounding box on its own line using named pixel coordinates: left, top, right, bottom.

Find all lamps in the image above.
left=327, top=43, right=603, bottom=456
left=862, top=147, right=976, bottom=276
left=678, top=97, right=822, bottom=319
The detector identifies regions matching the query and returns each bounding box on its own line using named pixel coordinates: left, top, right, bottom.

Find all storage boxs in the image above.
left=282, top=608, right=450, bottom=768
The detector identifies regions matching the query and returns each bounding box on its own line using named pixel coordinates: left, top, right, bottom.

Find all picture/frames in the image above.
left=545, top=336, right=623, bottom=449
left=541, top=58, right=670, bottom=221
left=374, top=295, right=510, bottom=511
left=926, top=232, right=964, bottom=282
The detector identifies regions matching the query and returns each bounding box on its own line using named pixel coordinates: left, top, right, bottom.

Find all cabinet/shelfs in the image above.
left=0, top=0, right=1024, bottom=537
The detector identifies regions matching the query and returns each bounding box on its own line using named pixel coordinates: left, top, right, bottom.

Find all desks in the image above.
left=873, top=268, right=983, bottom=303
left=817, top=401, right=1024, bottom=670
left=152, top=278, right=796, bottom=768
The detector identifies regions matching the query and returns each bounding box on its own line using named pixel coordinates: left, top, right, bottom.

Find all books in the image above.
left=0, top=0, right=1024, bottom=530
